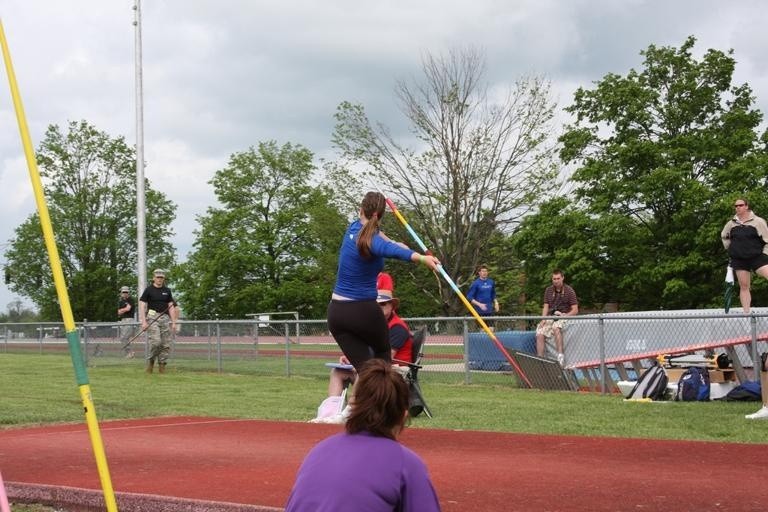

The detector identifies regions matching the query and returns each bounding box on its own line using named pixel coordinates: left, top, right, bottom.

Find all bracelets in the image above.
left=419, top=255, right=425, bottom=265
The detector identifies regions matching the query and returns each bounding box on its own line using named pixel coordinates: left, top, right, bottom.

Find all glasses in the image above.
left=733, top=204, right=747, bottom=207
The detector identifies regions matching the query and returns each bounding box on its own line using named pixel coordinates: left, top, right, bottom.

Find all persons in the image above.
left=466, top=265, right=499, bottom=332
left=285, top=357, right=441, bottom=512
left=535, top=267, right=579, bottom=368
left=744, top=350, right=768, bottom=419
left=115, top=284, right=136, bottom=359
left=720, top=198, right=768, bottom=312
left=138, top=267, right=180, bottom=375
left=310, top=300, right=413, bottom=425
left=325, top=189, right=441, bottom=376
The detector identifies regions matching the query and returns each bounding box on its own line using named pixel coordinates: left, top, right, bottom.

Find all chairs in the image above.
left=337, top=324, right=433, bottom=422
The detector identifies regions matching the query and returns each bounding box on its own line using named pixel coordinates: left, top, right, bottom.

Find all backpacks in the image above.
left=676, top=366, right=712, bottom=402
left=624, top=359, right=670, bottom=403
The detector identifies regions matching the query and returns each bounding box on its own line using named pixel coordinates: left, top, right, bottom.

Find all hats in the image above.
left=153, top=268, right=168, bottom=279
left=377, top=289, right=402, bottom=310
left=119, top=286, right=130, bottom=292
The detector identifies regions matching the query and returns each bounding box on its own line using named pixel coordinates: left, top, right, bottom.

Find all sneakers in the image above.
left=557, top=353, right=565, bottom=365
left=743, top=405, right=768, bottom=420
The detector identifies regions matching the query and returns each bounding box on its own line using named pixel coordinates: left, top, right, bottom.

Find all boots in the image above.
left=145, top=360, right=168, bottom=376
